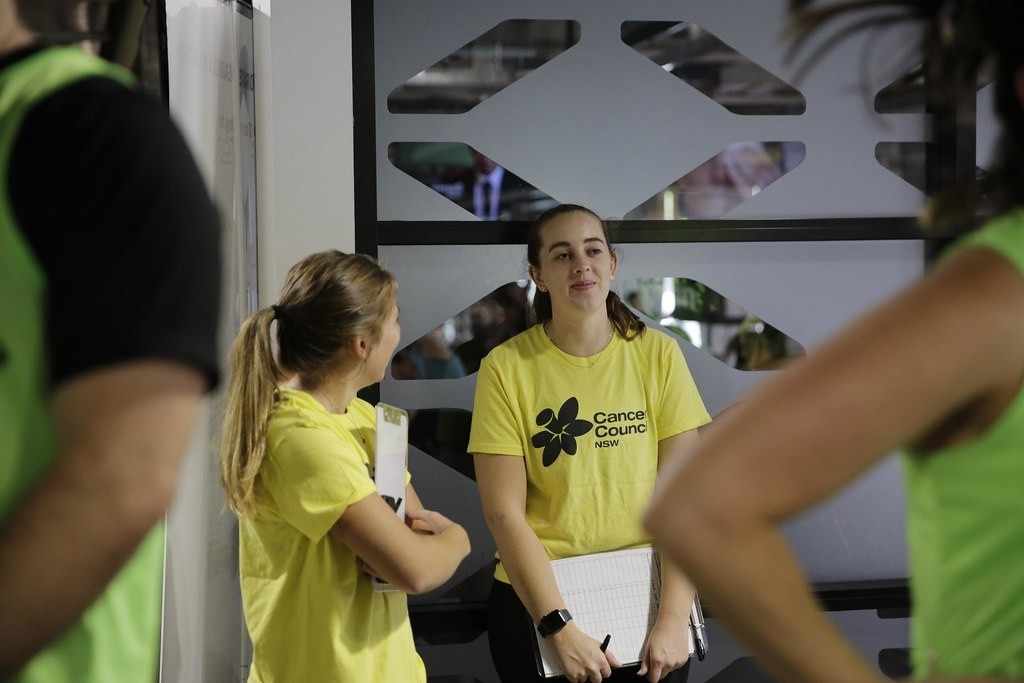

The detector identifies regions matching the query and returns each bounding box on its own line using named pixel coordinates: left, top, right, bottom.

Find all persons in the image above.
left=463, top=204, right=715, bottom=683
left=640, top=0, right=1024, bottom=683
left=0, top=0, right=228, bottom=681
left=218, top=245, right=472, bottom=683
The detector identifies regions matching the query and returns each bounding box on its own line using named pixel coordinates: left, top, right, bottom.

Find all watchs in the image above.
left=537, top=607, right=572, bottom=639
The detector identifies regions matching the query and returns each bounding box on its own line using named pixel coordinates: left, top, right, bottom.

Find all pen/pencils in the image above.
left=585, top=634, right=613, bottom=683
